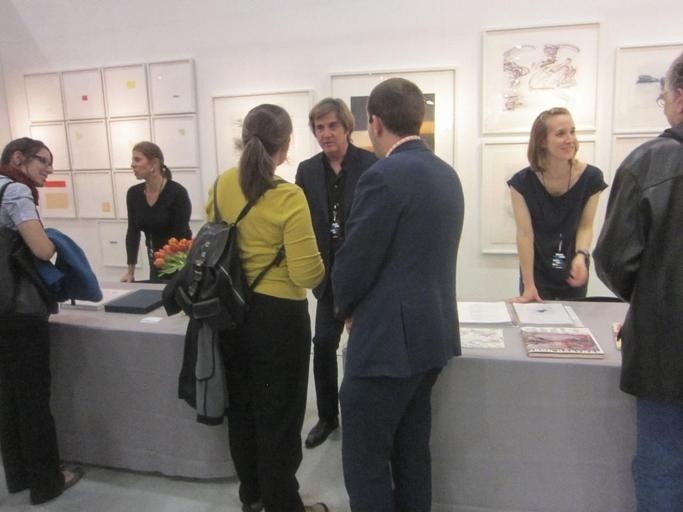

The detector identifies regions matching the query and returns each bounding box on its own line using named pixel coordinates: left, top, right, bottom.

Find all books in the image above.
left=456, top=300, right=622, bottom=359
left=104, top=288, right=163, bottom=314
left=60, top=288, right=130, bottom=311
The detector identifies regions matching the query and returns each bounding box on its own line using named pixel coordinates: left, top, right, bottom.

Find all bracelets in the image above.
left=575, top=249, right=588, bottom=264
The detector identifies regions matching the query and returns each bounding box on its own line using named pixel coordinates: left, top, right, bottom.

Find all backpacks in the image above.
left=174, top=175, right=289, bottom=332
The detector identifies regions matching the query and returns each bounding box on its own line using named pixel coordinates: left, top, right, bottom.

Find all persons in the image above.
left=506, top=107, right=608, bottom=303
left=0, top=137, right=83, bottom=506
left=205, top=103, right=328, bottom=512
left=120, top=142, right=193, bottom=283
left=592, top=52, right=683, bottom=512
left=294, top=98, right=379, bottom=448
left=331, top=77, right=464, bottom=512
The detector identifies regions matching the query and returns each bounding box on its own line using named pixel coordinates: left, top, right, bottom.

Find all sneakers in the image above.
left=242, top=493, right=329, bottom=511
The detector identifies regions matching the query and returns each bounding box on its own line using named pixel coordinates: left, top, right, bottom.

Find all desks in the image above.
left=45, top=277, right=237, bottom=482
left=428, top=298, right=640, bottom=510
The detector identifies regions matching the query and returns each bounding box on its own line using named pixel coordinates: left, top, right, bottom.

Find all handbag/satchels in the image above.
left=0, top=225, right=58, bottom=330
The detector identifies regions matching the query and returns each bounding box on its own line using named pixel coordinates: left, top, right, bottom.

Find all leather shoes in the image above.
left=64, top=466, right=84, bottom=490
left=306, top=416, right=338, bottom=448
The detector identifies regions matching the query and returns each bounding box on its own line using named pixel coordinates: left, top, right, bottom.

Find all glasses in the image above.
left=33, top=155, right=52, bottom=168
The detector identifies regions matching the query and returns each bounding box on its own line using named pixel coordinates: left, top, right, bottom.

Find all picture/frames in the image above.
left=610, top=43, right=681, bottom=136
left=325, top=68, right=458, bottom=179
left=211, top=88, right=318, bottom=188
left=478, top=138, right=597, bottom=258
left=608, top=133, right=664, bottom=198
left=479, top=15, right=603, bottom=137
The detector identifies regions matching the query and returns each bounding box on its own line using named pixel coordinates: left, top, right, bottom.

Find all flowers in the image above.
left=153, top=236, right=193, bottom=277
